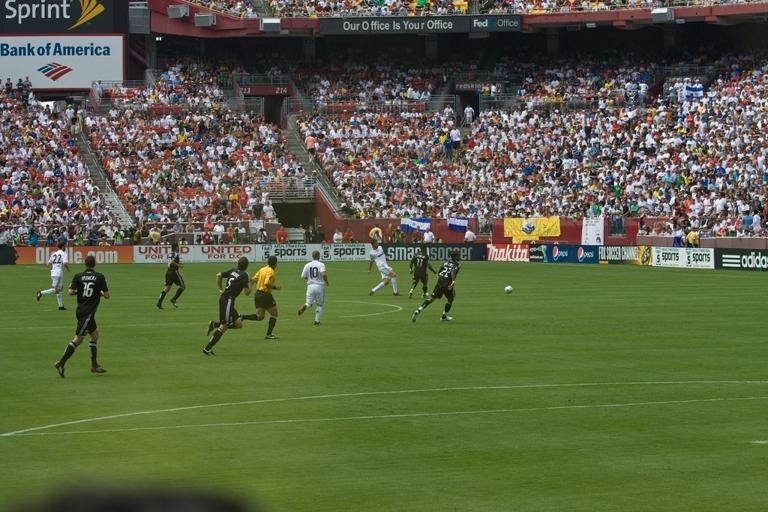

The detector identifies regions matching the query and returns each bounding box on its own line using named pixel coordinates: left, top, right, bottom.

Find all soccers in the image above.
left=504, top=285, right=513, bottom=293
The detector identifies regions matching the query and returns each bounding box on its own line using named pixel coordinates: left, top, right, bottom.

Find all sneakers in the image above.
left=89, top=365, right=107, bottom=373
left=202, top=303, right=322, bottom=356
left=408, top=290, right=429, bottom=299
left=59, top=306, right=67, bottom=310
left=55, top=361, right=65, bottom=377
left=440, top=315, right=453, bottom=320
left=410, top=310, right=419, bottom=322
left=368, top=287, right=372, bottom=295
left=393, top=292, right=399, bottom=296
left=156, top=301, right=164, bottom=309
left=170, top=299, right=178, bottom=308
left=36, top=290, right=42, bottom=301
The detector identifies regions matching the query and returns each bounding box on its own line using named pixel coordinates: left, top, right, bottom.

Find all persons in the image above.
left=368, top=239, right=403, bottom=296
left=298, top=251, right=329, bottom=326
left=37, top=242, right=70, bottom=310
left=239, top=256, right=281, bottom=339
left=200, top=257, right=257, bottom=357
left=54, top=256, right=110, bottom=378
left=408, top=245, right=437, bottom=299
left=411, top=251, right=462, bottom=323
left=156, top=243, right=185, bottom=309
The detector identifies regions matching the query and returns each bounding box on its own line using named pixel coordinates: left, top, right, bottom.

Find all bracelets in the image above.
left=273, top=286, right=277, bottom=289
